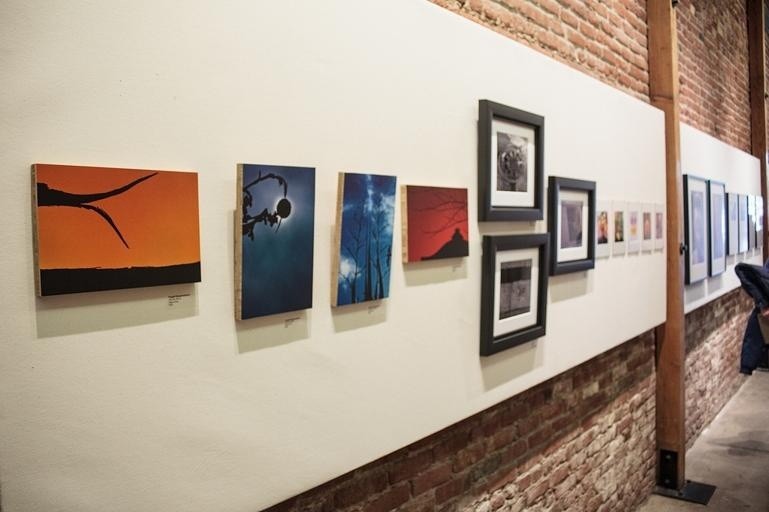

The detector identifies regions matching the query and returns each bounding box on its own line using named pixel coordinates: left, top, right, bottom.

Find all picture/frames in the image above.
left=682, top=173, right=730, bottom=286
left=475, top=99, right=596, bottom=358
left=593, top=200, right=666, bottom=261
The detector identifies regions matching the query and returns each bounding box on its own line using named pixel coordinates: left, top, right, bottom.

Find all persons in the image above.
left=735, top=258, right=769, bottom=375
